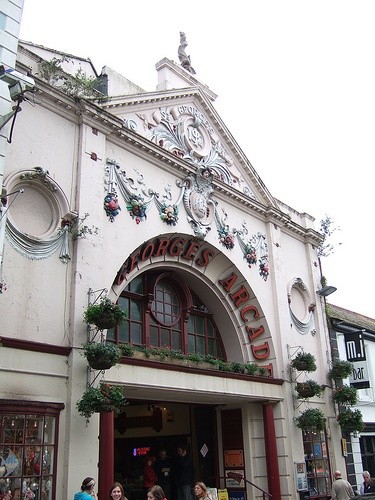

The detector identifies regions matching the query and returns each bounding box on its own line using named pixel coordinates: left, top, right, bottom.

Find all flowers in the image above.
left=106, top=198, right=269, bottom=275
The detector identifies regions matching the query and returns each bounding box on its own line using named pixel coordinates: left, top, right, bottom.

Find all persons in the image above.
left=172, top=446, right=195, bottom=500
left=109, top=481, right=129, bottom=500
left=74, top=477, right=98, bottom=500
left=193, top=481, right=213, bottom=500
left=143, top=448, right=172, bottom=500
left=361, top=470, right=375, bottom=495
left=329, top=470, right=355, bottom=500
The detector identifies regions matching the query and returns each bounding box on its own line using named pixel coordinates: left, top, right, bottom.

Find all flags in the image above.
left=343, top=330, right=371, bottom=390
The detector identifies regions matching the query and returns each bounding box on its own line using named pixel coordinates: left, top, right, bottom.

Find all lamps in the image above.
left=316, top=286, right=337, bottom=297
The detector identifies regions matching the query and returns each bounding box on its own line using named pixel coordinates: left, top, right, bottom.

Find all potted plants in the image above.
left=76, top=298, right=365, bottom=437
